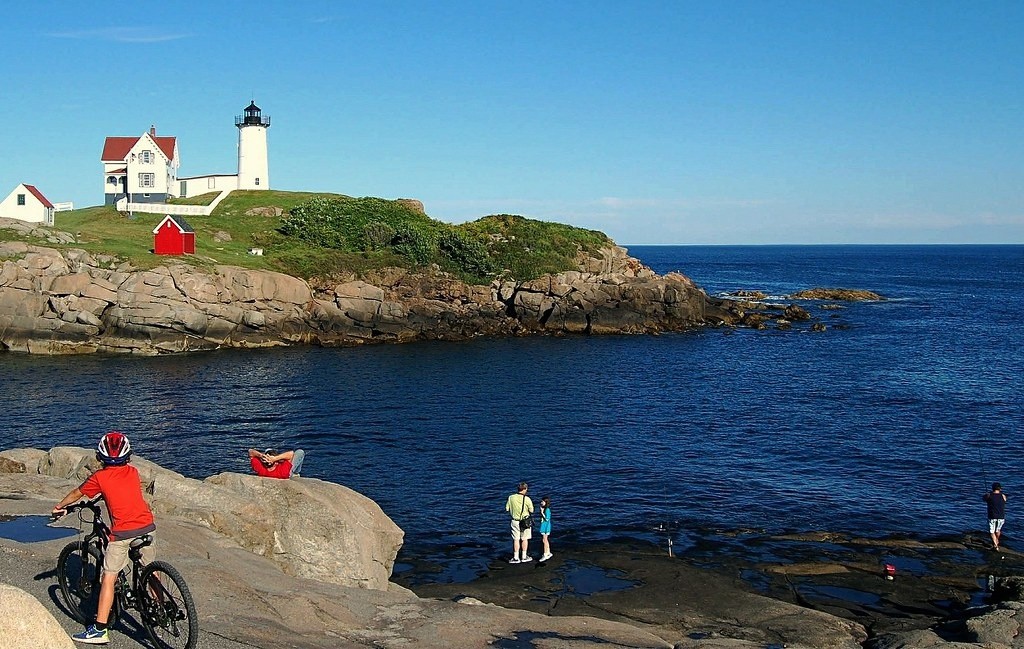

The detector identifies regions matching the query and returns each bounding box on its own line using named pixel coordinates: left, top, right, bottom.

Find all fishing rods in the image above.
left=661, top=480, right=676, bottom=560
left=972, top=419, right=993, bottom=496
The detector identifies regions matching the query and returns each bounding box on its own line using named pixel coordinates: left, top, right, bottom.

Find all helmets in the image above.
left=95, top=430, right=133, bottom=466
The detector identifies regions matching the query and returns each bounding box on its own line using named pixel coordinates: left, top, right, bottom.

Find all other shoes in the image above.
left=540, top=553, right=553, bottom=562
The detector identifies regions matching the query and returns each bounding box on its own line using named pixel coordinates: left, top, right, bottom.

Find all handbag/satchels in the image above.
left=519, top=516, right=535, bottom=532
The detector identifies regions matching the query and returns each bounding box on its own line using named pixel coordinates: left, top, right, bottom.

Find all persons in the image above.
left=52, top=430, right=158, bottom=644
left=982, top=482, right=1007, bottom=551
left=248, top=448, right=306, bottom=479
left=538, top=496, right=554, bottom=562
left=505, top=482, right=535, bottom=564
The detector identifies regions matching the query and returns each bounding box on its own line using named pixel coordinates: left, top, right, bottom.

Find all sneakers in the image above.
left=71, top=624, right=110, bottom=645
left=522, top=556, right=532, bottom=562
left=509, top=557, right=521, bottom=563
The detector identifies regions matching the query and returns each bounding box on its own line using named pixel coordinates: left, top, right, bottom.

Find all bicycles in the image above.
left=49, top=493, right=200, bottom=649
